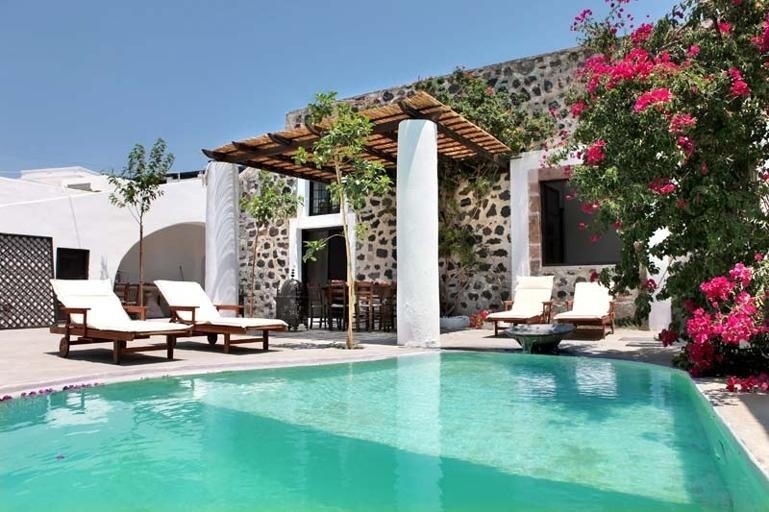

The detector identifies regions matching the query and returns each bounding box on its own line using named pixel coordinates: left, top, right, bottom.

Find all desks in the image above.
left=123, top=302, right=151, bottom=322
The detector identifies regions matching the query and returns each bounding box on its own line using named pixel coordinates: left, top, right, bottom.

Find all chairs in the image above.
left=111, top=279, right=158, bottom=307
left=482, top=273, right=557, bottom=337
left=550, top=280, right=619, bottom=341
left=294, top=275, right=398, bottom=334
left=47, top=274, right=191, bottom=366
left=151, top=278, right=292, bottom=358
